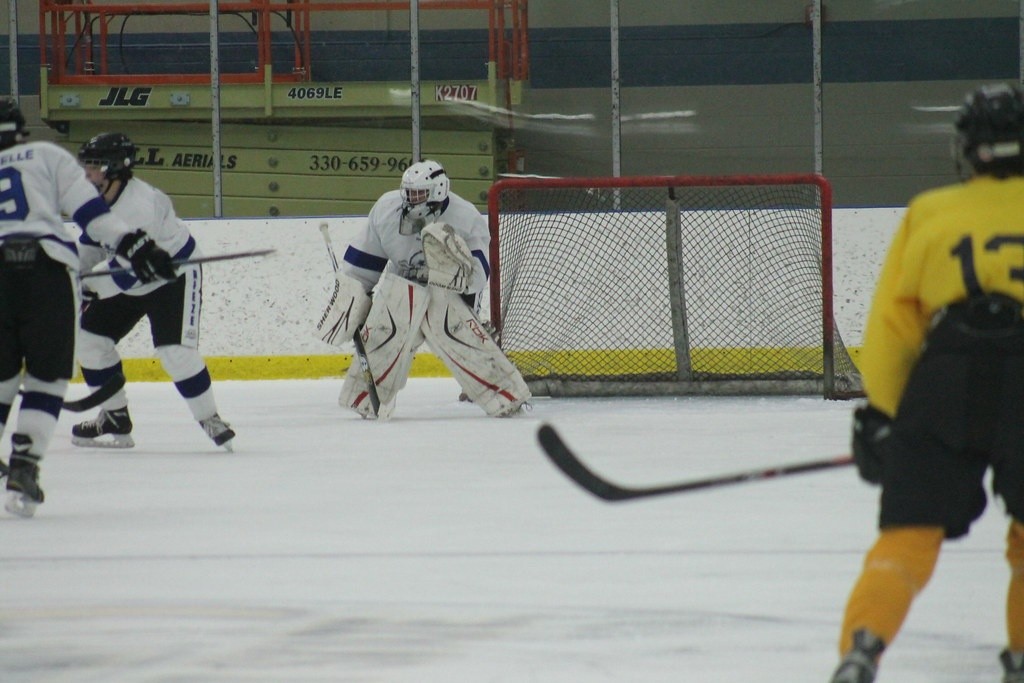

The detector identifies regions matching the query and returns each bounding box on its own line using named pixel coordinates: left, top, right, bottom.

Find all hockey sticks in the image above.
left=10, top=372, right=126, bottom=414
left=318, top=219, right=406, bottom=424
left=73, top=248, right=279, bottom=281
left=533, top=420, right=857, bottom=505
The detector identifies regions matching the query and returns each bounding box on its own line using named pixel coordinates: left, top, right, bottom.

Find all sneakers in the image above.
left=71, top=405, right=135, bottom=448
left=4, top=434, right=45, bottom=518
left=0, top=461, right=9, bottom=479
left=199, top=413, right=235, bottom=453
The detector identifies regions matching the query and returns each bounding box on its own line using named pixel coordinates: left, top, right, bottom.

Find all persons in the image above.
left=0, top=95, right=179, bottom=518
left=827, top=83, right=1024, bottom=683
left=313, top=160, right=531, bottom=419
left=65, top=132, right=236, bottom=457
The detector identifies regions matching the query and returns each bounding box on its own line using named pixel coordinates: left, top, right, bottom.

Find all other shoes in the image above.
left=1001, top=649, right=1024, bottom=683
left=830, top=629, right=885, bottom=683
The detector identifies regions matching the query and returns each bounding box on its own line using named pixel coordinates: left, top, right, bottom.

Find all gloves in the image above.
left=116, top=228, right=177, bottom=285
left=81, top=291, right=97, bottom=312
left=853, top=406, right=892, bottom=484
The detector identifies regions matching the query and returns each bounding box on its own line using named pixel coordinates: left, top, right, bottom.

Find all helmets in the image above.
left=79, top=133, right=136, bottom=161
left=0, top=96, right=26, bottom=123
left=951, top=81, right=1024, bottom=179
left=400, top=160, right=449, bottom=219
